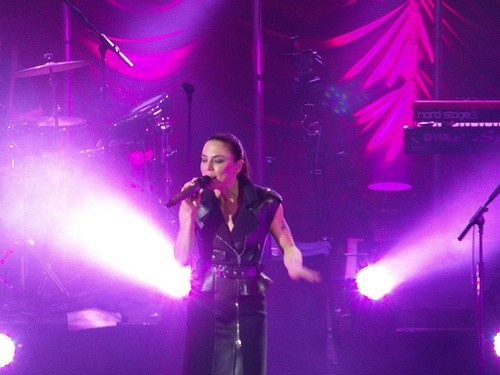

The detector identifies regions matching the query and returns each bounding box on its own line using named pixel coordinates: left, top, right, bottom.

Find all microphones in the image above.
left=165, top=176, right=211, bottom=209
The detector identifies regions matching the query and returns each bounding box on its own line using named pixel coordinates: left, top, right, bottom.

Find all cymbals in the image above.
left=28, top=114, right=88, bottom=129
left=14, top=59, right=89, bottom=79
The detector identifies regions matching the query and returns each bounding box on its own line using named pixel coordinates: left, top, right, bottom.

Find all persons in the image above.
left=173, top=133, right=321, bottom=375
